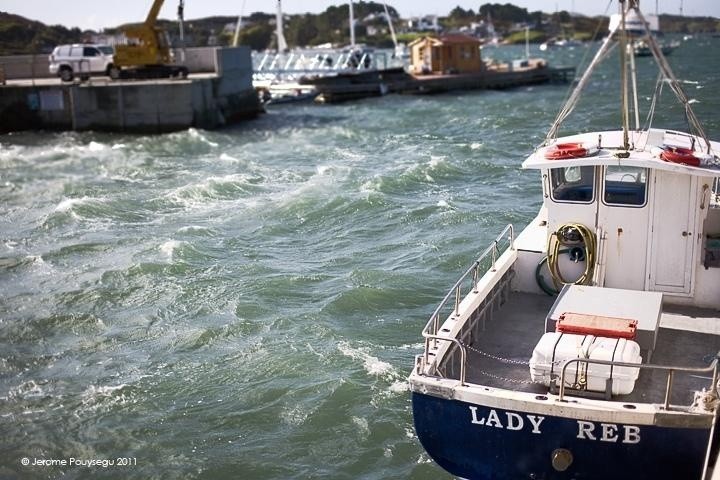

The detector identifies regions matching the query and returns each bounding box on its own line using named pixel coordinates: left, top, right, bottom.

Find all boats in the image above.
left=408, top=2, right=719, bottom=480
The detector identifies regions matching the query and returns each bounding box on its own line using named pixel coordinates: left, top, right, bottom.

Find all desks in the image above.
left=544, top=282, right=664, bottom=354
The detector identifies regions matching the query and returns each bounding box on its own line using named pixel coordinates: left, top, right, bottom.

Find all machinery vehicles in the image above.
left=107, top=0, right=188, bottom=80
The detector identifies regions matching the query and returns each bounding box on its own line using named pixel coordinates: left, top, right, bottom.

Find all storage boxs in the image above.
left=529, top=313, right=644, bottom=394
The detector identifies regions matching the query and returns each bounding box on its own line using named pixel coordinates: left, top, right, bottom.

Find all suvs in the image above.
left=48, top=44, right=115, bottom=81
